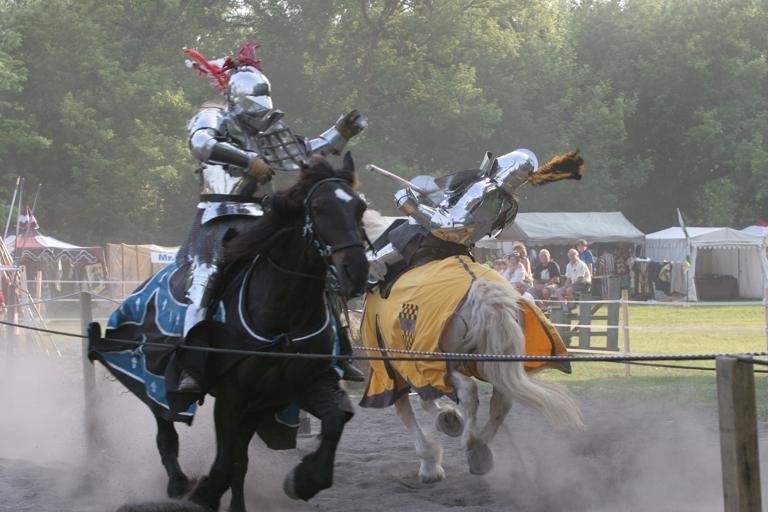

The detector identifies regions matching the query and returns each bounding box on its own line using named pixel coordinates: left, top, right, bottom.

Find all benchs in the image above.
left=519, top=276, right=604, bottom=332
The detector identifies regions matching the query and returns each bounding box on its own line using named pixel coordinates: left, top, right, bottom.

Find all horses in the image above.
left=90, top=151, right=368, bottom=512
left=362, top=210, right=587, bottom=482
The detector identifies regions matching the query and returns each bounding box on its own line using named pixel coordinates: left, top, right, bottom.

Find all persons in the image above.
left=369, top=143, right=541, bottom=291
left=165, top=65, right=368, bottom=416
left=470, top=236, right=650, bottom=316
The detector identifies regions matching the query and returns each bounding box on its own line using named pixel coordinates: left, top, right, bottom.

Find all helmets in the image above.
left=224, top=65, right=285, bottom=132
left=480, top=148, right=538, bottom=195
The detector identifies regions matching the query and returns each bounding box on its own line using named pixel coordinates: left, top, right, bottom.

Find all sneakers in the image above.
left=336, top=358, right=365, bottom=382
left=178, top=371, right=202, bottom=393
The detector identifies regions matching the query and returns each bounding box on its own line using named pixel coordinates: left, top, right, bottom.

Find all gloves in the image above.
left=335, top=110, right=364, bottom=141
left=248, top=157, right=275, bottom=187
